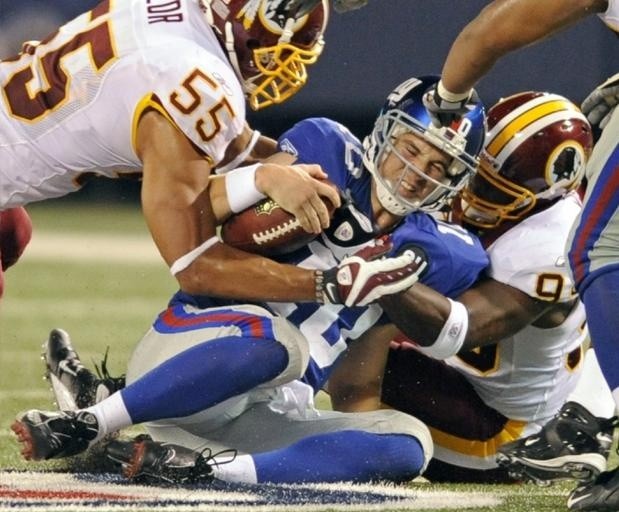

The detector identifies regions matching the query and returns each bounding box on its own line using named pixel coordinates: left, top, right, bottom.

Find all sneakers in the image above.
left=42, top=328, right=97, bottom=412
left=496, top=420, right=612, bottom=483
left=12, top=409, right=98, bottom=462
left=566, top=469, right=618, bottom=511
left=106, top=439, right=206, bottom=485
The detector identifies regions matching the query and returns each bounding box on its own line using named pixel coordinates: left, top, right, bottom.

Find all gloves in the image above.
left=422, top=81, right=472, bottom=129
left=581, top=72, right=619, bottom=130
left=322, top=234, right=418, bottom=308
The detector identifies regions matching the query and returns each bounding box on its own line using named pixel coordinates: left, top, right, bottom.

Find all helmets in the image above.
left=452, top=91, right=593, bottom=228
left=198, top=0, right=329, bottom=110
left=364, top=75, right=487, bottom=217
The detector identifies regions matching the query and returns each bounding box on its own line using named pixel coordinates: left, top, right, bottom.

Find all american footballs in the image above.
left=220, top=177, right=342, bottom=259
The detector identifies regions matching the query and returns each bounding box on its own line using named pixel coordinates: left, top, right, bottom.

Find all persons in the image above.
left=423, top=1, right=619, bottom=512
left=11, top=74, right=486, bottom=487
left=41, top=91, right=594, bottom=482
left=0, top=0, right=429, bottom=309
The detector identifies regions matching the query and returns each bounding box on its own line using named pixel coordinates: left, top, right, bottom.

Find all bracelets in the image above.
left=435, top=79, right=474, bottom=102
left=226, top=163, right=268, bottom=216
left=419, top=294, right=470, bottom=362
